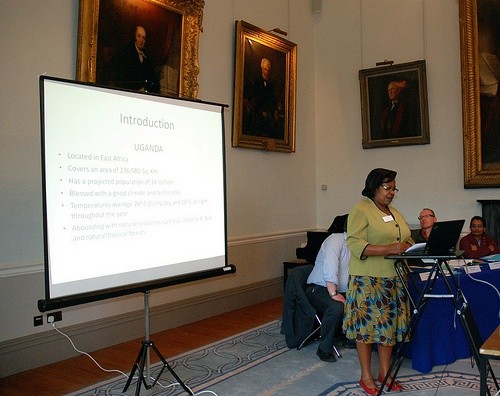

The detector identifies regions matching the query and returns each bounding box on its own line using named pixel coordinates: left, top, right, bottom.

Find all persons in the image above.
left=415, top=209, right=437, bottom=244
left=459, top=216, right=500, bottom=259
left=342, top=168, right=415, bottom=396
left=304, top=213, right=350, bottom=362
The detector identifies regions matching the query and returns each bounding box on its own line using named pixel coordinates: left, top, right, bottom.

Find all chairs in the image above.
left=280, top=265, right=342, bottom=358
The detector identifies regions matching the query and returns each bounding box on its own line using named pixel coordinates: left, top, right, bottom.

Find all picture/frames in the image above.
left=75, top=0, right=205, bottom=100
left=358, top=60, right=430, bottom=150
left=232, top=20, right=297, bottom=153
left=459, top=0, right=500, bottom=189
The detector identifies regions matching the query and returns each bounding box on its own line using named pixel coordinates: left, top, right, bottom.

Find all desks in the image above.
left=377, top=255, right=500, bottom=396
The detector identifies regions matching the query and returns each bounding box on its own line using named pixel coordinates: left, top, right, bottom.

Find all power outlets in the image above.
left=47, top=311, right=62, bottom=323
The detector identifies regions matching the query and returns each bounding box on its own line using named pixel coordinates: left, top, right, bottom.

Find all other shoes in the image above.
left=359, top=379, right=380, bottom=396
left=342, top=339, right=356, bottom=347
left=378, top=375, right=402, bottom=391
left=316, top=346, right=336, bottom=362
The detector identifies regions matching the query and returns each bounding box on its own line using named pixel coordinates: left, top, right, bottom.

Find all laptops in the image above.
left=401, top=220, right=464, bottom=255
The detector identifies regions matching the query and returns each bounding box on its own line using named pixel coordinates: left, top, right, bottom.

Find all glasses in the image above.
left=418, top=214, right=434, bottom=219
left=381, top=185, right=398, bottom=193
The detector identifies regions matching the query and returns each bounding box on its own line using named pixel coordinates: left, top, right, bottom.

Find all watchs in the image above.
left=330, top=290, right=338, bottom=297
